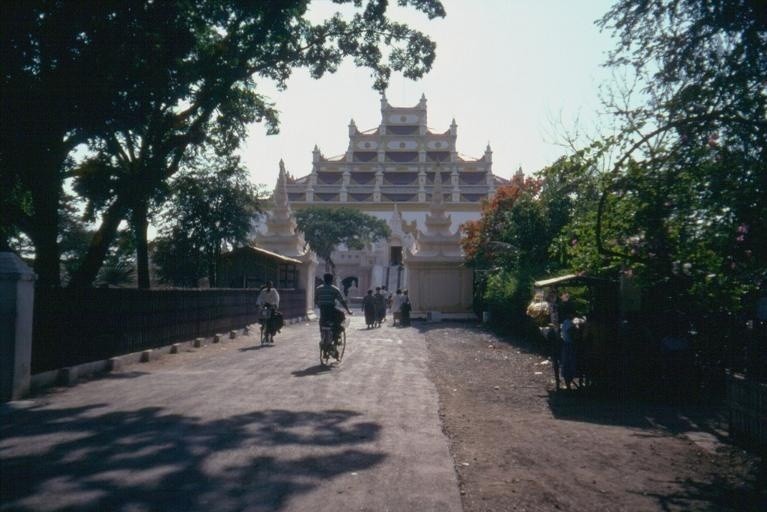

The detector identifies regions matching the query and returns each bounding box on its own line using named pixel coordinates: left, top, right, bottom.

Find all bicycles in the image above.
left=258, top=304, right=276, bottom=344
left=318, top=310, right=351, bottom=367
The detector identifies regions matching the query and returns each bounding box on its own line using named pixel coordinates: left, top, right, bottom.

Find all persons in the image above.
left=255, top=279, right=280, bottom=342
left=561, top=305, right=606, bottom=389
left=361, top=285, right=412, bottom=329
left=314, top=272, right=353, bottom=358
